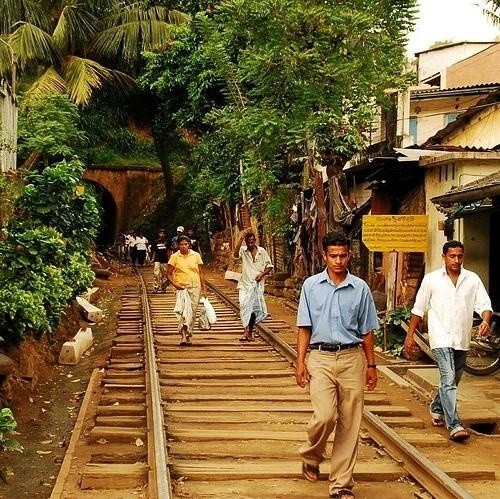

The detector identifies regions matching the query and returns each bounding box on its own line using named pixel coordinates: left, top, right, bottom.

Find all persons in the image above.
left=165, top=234, right=208, bottom=347
left=231, top=228, right=275, bottom=342
left=294, top=231, right=381, bottom=499
left=405, top=239, right=494, bottom=441
left=125, top=225, right=201, bottom=293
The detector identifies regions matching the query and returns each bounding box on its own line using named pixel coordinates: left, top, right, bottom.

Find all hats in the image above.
left=177, top=226, right=184, bottom=232
left=158, top=228, right=166, bottom=232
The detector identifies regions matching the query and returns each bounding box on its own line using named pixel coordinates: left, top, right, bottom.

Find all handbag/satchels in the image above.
left=202, top=299, right=219, bottom=324
left=197, top=307, right=212, bottom=331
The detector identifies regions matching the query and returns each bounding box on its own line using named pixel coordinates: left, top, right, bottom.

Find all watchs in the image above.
left=367, top=364, right=376, bottom=369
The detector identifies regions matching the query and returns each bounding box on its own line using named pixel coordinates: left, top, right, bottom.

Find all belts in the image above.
left=309, top=343, right=361, bottom=353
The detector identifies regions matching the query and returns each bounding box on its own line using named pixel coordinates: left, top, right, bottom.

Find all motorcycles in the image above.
left=464, top=311, right=500, bottom=377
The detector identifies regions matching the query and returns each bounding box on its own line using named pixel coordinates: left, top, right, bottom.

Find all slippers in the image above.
left=331, top=488, right=356, bottom=499
left=180, top=341, right=193, bottom=345
left=300, top=461, right=321, bottom=483
left=432, top=417, right=444, bottom=427
left=239, top=333, right=255, bottom=342
left=449, top=432, right=471, bottom=441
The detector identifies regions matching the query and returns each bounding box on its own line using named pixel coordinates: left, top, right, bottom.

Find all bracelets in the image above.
left=483, top=321, right=488, bottom=326
